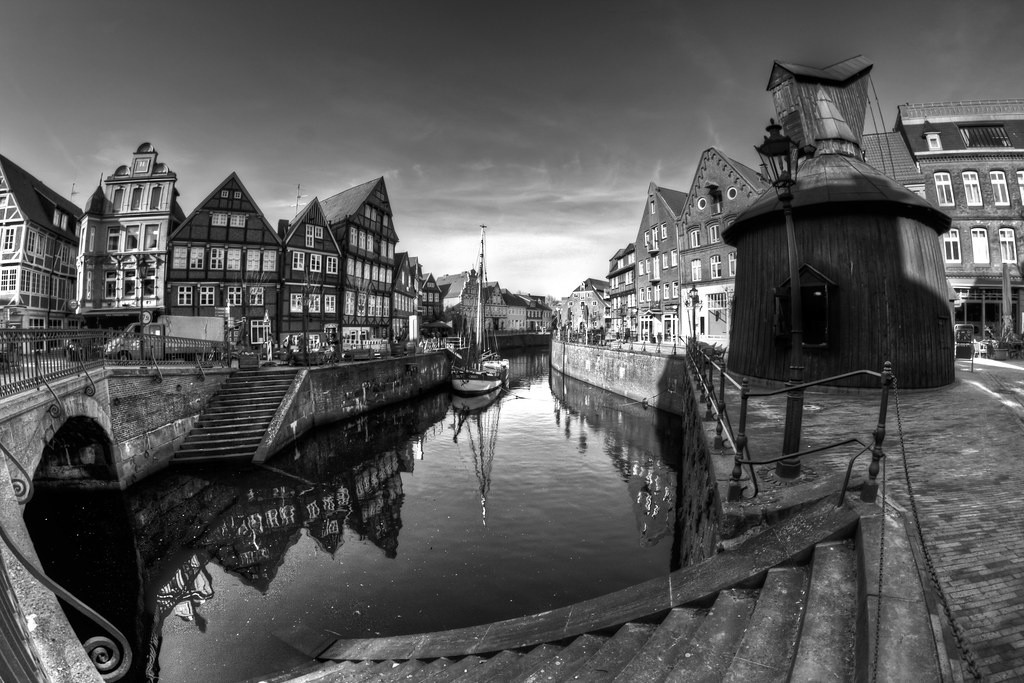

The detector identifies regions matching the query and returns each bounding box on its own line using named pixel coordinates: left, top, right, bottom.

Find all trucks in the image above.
left=103, top=314, right=227, bottom=362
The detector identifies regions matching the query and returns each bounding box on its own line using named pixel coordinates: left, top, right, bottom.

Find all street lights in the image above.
left=689, top=284, right=699, bottom=358
left=757, top=117, right=806, bottom=474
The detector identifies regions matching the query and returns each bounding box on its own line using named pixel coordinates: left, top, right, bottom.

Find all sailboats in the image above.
left=451, top=223, right=509, bottom=391
left=450, top=380, right=509, bottom=526
